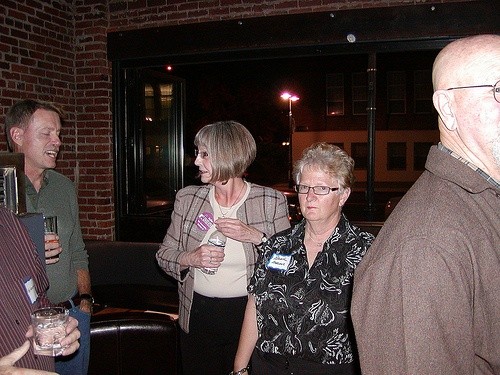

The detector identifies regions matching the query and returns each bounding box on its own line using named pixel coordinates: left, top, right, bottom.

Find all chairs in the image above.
left=85, top=311, right=182, bottom=375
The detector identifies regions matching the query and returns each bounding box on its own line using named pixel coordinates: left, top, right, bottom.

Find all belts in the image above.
left=53, top=295, right=81, bottom=314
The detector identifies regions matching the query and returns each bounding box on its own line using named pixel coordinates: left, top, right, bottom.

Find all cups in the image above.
left=30, top=306, right=69, bottom=357
left=42, top=215, right=60, bottom=264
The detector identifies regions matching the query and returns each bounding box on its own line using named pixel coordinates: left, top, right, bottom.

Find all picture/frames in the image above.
left=413, top=69, right=434, bottom=115
left=328, top=142, right=344, bottom=150
left=324, top=71, right=345, bottom=117
left=386, top=141, right=407, bottom=171
left=351, top=71, right=369, bottom=116
left=386, top=70, right=407, bottom=116
left=351, top=142, right=369, bottom=171
left=413, top=141, right=433, bottom=171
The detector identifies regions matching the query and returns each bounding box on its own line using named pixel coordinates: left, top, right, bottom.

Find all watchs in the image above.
left=79, top=293, right=94, bottom=306
left=254, top=232, right=267, bottom=246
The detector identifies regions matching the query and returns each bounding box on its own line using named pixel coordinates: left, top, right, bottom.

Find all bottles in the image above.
left=201, top=230, right=226, bottom=275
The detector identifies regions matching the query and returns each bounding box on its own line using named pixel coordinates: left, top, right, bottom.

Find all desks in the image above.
left=94, top=307, right=179, bottom=321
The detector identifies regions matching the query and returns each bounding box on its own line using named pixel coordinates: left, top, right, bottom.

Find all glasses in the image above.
left=446, top=79, right=500, bottom=103
left=293, top=184, right=339, bottom=195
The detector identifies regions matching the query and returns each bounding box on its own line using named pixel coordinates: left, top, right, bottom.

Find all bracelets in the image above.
left=229, top=365, right=250, bottom=375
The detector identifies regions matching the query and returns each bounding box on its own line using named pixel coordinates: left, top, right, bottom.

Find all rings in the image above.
left=208, top=257, right=212, bottom=263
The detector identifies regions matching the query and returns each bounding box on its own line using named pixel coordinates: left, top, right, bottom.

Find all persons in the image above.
left=229, top=142, right=376, bottom=375
left=0, top=98, right=94, bottom=375
left=155, top=120, right=293, bottom=375
left=350, top=35, right=500, bottom=375
left=0, top=208, right=81, bottom=375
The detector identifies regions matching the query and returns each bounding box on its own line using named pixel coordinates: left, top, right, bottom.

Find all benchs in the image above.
left=83, top=238, right=179, bottom=314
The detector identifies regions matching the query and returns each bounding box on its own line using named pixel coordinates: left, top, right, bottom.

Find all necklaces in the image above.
left=305, top=229, right=329, bottom=246
left=215, top=182, right=245, bottom=218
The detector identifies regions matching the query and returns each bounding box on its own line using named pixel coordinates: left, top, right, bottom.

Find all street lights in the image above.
left=279, top=92, right=299, bottom=184
